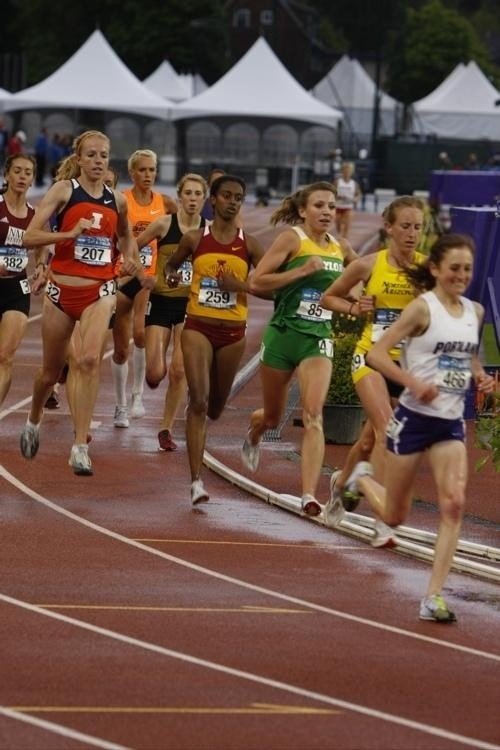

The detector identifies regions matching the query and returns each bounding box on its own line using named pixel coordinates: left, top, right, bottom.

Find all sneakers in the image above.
left=190, top=481, right=209, bottom=504
left=372, top=520, right=398, bottom=549
left=301, top=494, right=321, bottom=517
left=130, top=392, right=145, bottom=418
left=114, top=406, right=128, bottom=428
left=67, top=431, right=94, bottom=476
left=241, top=424, right=262, bottom=472
left=21, top=410, right=44, bottom=458
left=157, top=430, right=178, bottom=451
left=339, top=461, right=373, bottom=512
left=43, top=392, right=60, bottom=410
left=323, top=470, right=346, bottom=526
left=419, top=595, right=455, bottom=622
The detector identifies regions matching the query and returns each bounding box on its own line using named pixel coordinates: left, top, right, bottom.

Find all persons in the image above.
left=340, top=234, right=500, bottom=621
left=440, top=153, right=498, bottom=169
left=34, top=125, right=51, bottom=187
left=1, top=130, right=360, bottom=515
left=320, top=197, right=429, bottom=547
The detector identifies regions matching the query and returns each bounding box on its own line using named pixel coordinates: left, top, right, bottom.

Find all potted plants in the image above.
left=315, top=322, right=370, bottom=448
